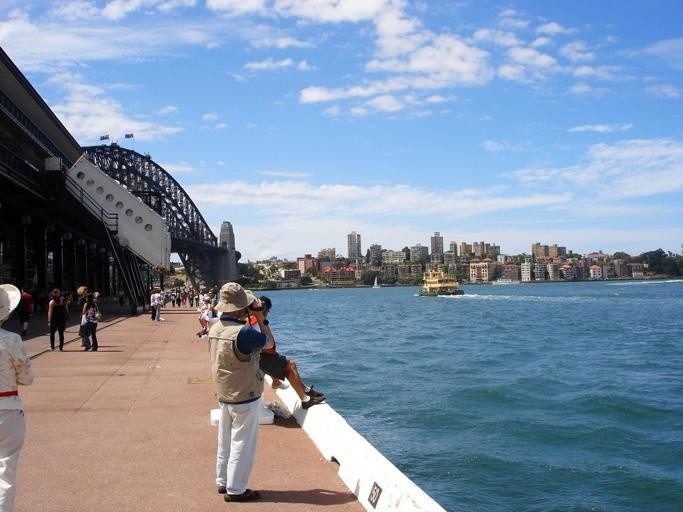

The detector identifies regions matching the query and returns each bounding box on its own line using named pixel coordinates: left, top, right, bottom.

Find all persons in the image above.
left=16, top=285, right=102, bottom=353
left=118, top=287, right=125, bottom=307
left=246, top=296, right=327, bottom=409
left=150, top=286, right=216, bottom=338
left=207, top=282, right=274, bottom=502
left=0, top=284, right=35, bottom=512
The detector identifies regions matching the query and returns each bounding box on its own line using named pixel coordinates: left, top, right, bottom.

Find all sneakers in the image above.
left=218, top=486, right=227, bottom=493
left=271, top=382, right=289, bottom=389
left=224, top=489, right=261, bottom=502
left=301, top=385, right=326, bottom=410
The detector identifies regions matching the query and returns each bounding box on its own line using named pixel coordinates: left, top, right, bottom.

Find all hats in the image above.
left=0, top=284, right=22, bottom=321
left=213, top=282, right=255, bottom=313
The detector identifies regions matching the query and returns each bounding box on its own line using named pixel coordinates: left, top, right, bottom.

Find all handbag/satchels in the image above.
left=88, top=309, right=94, bottom=319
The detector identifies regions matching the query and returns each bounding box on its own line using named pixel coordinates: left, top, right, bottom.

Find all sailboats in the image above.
left=372, top=276, right=380, bottom=288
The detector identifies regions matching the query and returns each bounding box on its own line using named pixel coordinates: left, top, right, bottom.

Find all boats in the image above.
left=419, top=265, right=462, bottom=296
